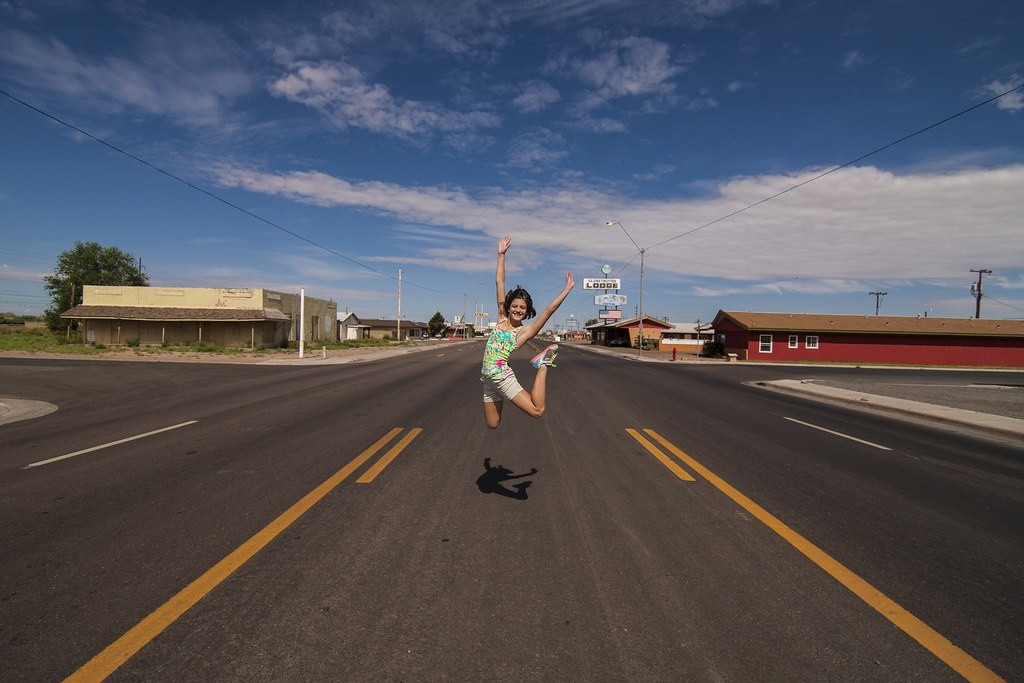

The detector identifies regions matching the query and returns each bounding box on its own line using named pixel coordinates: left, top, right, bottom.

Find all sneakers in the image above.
left=528, top=344, right=560, bottom=370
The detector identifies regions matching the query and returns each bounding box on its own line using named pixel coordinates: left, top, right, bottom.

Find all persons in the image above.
left=480, top=236, right=576, bottom=429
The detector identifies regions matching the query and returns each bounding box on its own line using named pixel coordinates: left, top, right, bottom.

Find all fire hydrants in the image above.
left=672, top=347, right=677, bottom=361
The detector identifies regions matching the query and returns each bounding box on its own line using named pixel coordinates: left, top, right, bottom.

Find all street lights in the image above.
left=606, top=221, right=645, bottom=358
